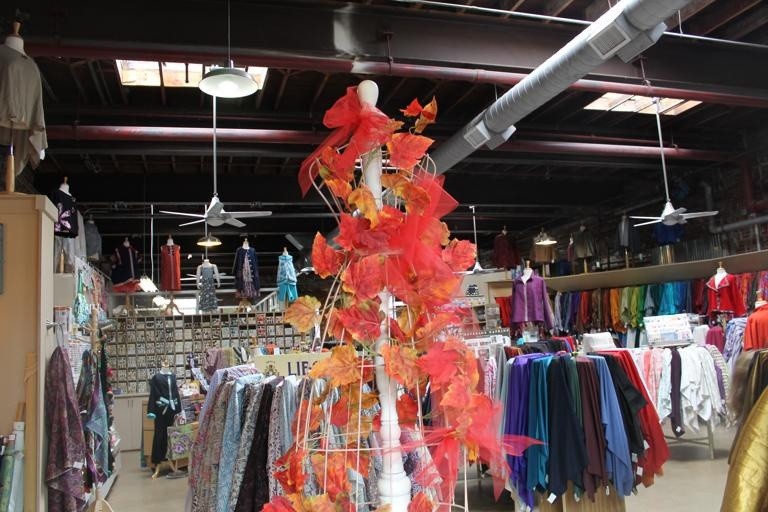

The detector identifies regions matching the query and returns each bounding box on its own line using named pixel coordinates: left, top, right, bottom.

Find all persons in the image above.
left=66, top=208, right=299, bottom=310
left=741, top=298, right=767, bottom=350
left=510, top=266, right=554, bottom=332
left=706, top=266, right=744, bottom=324
left=54, top=182, right=79, bottom=273
left=0, top=35, right=48, bottom=177
left=490, top=209, right=685, bottom=268
left=147, top=362, right=187, bottom=479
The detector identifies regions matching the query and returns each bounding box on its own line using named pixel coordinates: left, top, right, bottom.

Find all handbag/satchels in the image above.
left=164, top=414, right=201, bottom=461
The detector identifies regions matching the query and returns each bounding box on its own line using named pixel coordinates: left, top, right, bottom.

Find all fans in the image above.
left=452, top=207, right=504, bottom=274
left=627, top=93, right=721, bottom=230
left=157, top=97, right=273, bottom=230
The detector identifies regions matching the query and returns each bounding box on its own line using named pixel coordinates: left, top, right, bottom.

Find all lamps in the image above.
left=195, top=231, right=223, bottom=249
left=196, top=0, right=259, bottom=101
